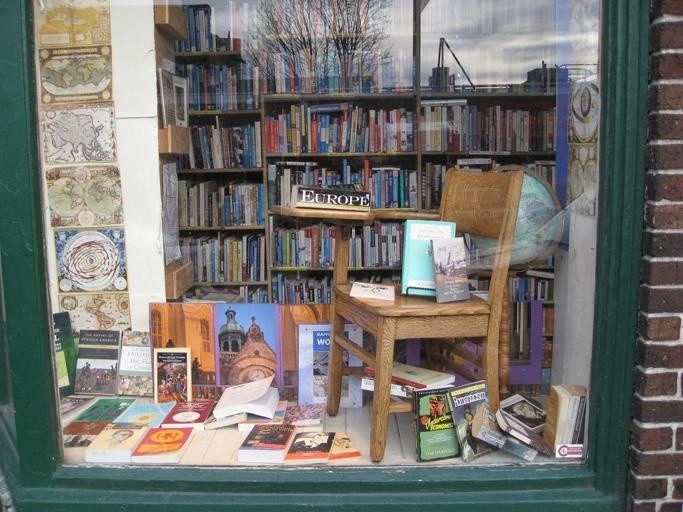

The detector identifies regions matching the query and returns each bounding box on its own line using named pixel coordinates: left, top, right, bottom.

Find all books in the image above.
left=161, top=0, right=586, bottom=392
left=53, top=303, right=586, bottom=467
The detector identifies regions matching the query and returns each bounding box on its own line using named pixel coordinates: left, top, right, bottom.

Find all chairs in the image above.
left=320, top=166, right=528, bottom=465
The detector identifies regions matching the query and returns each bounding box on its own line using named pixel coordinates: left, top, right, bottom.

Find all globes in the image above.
left=463, top=164, right=564, bottom=408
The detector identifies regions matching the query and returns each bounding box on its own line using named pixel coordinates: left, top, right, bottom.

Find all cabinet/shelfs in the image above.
left=150, top=2, right=556, bottom=377
